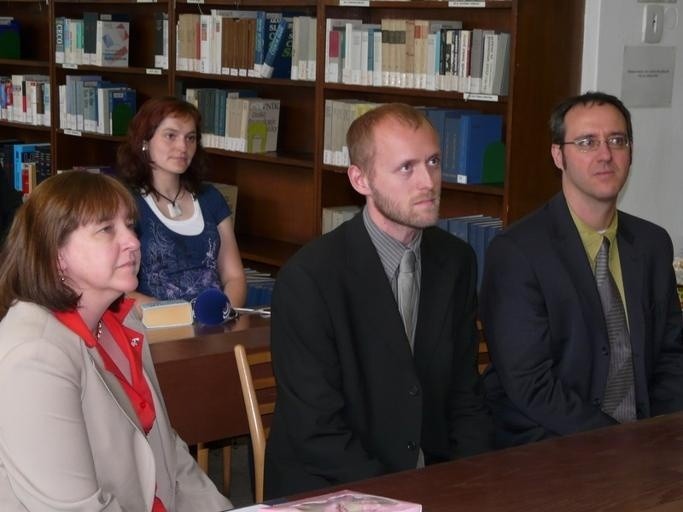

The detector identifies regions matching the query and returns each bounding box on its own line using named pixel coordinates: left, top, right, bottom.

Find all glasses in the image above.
left=563, top=136, right=629, bottom=149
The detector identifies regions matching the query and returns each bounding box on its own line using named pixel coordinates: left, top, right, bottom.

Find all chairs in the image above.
left=235, top=314, right=273, bottom=502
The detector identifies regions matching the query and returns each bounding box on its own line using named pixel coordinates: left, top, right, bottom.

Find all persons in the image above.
left=260, top=103, right=494, bottom=504
left=0, top=168, right=233, bottom=510
left=472, top=92, right=682, bottom=455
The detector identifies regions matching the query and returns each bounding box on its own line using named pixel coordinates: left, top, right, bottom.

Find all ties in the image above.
left=595, top=235, right=637, bottom=423
left=397, top=249, right=420, bottom=356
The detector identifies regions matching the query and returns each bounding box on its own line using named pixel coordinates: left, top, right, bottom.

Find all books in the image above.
left=258, top=489, right=422, bottom=512
left=1, top=4, right=508, bottom=314
left=139, top=298, right=195, bottom=329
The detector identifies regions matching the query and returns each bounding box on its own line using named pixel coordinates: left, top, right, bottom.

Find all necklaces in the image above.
left=91, top=318, right=104, bottom=340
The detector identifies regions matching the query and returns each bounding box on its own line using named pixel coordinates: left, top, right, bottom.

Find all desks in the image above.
left=232, top=410, right=682, bottom=510
